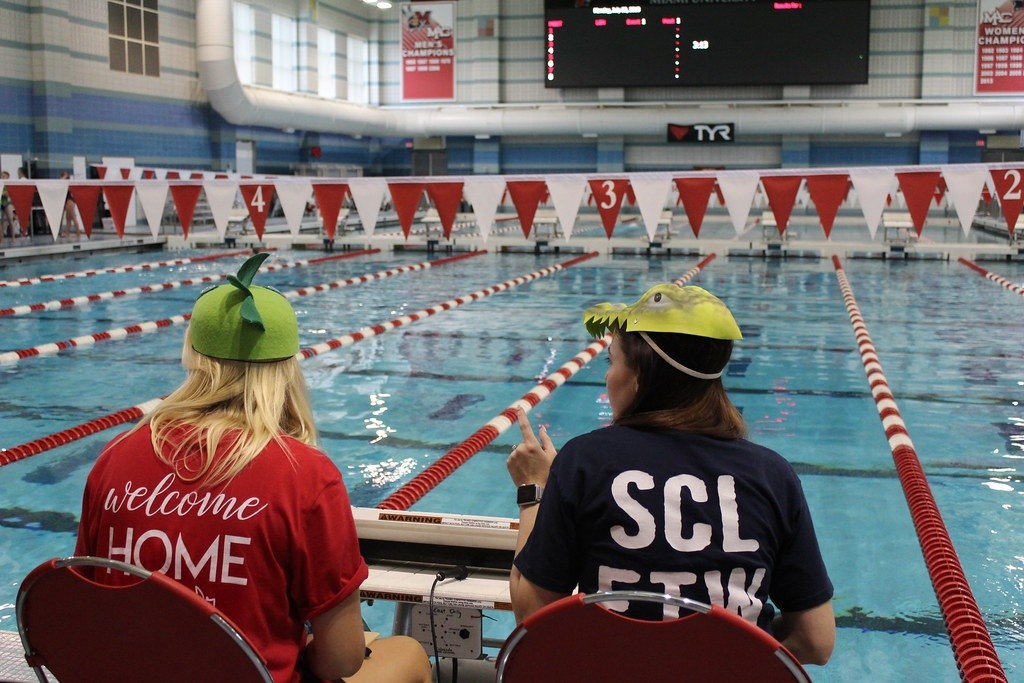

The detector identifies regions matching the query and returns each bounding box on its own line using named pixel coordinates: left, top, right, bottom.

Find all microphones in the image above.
left=436, top=566, right=468, bottom=581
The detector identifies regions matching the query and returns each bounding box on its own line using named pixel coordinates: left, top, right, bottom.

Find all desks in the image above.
left=357, top=566, right=515, bottom=648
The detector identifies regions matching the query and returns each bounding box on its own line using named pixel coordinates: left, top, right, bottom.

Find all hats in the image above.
left=190, top=253, right=300, bottom=364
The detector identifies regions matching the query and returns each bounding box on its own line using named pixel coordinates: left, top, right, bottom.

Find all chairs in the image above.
left=14, top=556, right=275, bottom=683
left=494, top=590, right=815, bottom=683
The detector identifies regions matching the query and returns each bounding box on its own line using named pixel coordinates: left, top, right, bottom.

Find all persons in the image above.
left=504, top=287, right=837, bottom=682
left=73, top=280, right=434, bottom=683
left=0, top=166, right=83, bottom=245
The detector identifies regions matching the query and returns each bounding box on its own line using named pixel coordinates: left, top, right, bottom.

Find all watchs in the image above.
left=515, top=482, right=544, bottom=506
